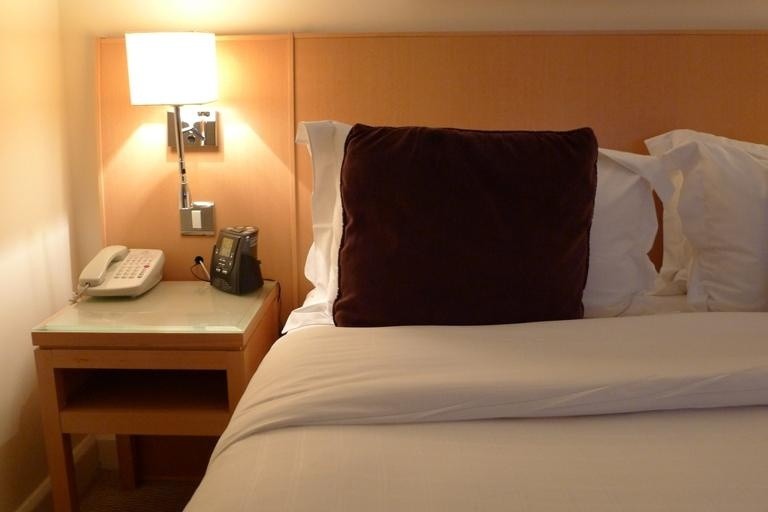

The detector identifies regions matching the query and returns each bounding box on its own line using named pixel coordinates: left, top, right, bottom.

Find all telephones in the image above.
left=77, top=245, right=165, bottom=298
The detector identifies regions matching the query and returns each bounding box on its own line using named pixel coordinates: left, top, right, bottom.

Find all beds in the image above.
left=98, top=30, right=768, bottom=510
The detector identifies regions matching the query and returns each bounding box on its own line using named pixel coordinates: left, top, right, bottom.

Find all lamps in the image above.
left=124, top=34, right=217, bottom=210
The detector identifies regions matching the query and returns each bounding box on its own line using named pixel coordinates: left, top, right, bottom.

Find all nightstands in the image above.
left=32, top=281, right=280, bottom=511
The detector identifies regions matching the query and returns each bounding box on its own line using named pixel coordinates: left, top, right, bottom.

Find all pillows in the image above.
left=664, top=139, right=768, bottom=313
left=283, top=117, right=673, bottom=331
left=642, top=126, right=768, bottom=297
left=333, top=121, right=599, bottom=329
